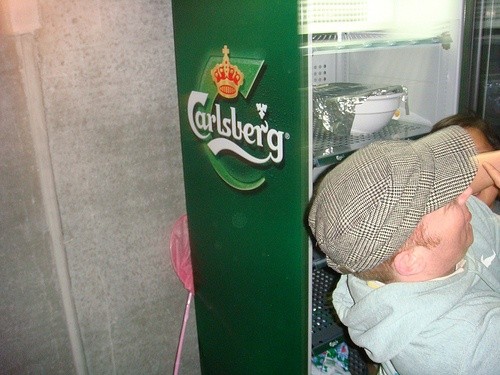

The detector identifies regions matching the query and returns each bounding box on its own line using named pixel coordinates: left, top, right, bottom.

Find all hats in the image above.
left=309, top=125, right=478, bottom=274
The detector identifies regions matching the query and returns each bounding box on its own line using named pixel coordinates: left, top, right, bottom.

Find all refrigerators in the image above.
left=173, top=0, right=466, bottom=375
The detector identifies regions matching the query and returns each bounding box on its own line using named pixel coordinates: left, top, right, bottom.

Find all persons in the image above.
left=307, top=127, right=499, bottom=374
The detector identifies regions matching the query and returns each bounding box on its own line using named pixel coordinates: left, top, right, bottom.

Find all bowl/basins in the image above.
left=314, top=83, right=404, bottom=134
